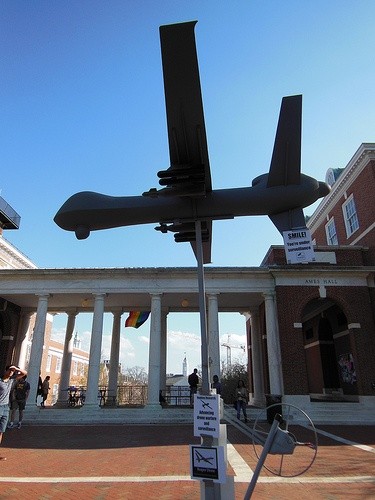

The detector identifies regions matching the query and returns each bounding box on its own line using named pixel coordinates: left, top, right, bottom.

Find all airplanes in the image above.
left=53, top=20, right=333, bottom=264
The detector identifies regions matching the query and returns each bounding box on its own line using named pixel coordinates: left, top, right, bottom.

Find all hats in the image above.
left=23, top=375, right=27, bottom=381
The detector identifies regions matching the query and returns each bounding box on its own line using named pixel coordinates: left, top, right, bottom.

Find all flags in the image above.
left=125, top=311, right=151, bottom=327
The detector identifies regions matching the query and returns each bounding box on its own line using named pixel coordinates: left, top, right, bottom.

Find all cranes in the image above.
left=222, top=335, right=245, bottom=371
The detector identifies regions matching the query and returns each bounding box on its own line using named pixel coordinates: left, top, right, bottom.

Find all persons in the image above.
left=0, top=365, right=51, bottom=461
left=211, top=375, right=224, bottom=396
left=234, top=380, right=247, bottom=423
left=188, top=368, right=200, bottom=408
left=67, top=387, right=168, bottom=407
left=338, top=356, right=353, bottom=385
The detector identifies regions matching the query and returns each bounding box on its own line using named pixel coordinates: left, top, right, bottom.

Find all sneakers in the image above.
left=7, top=424, right=13, bottom=429
left=17, top=424, right=21, bottom=430
